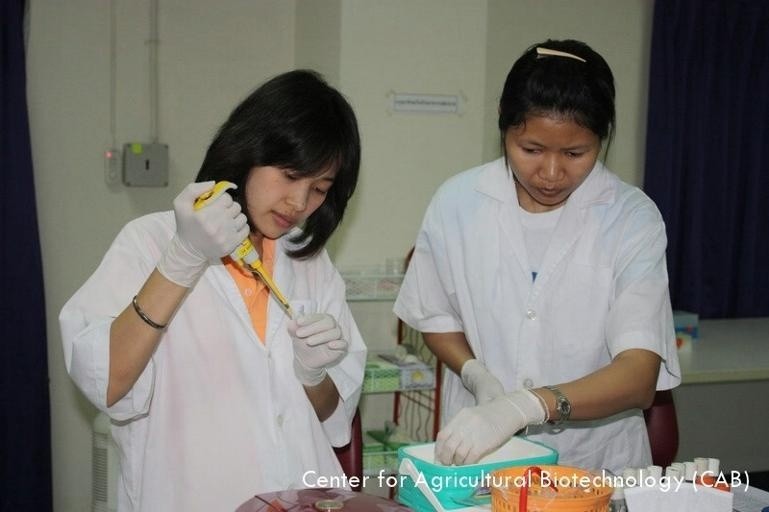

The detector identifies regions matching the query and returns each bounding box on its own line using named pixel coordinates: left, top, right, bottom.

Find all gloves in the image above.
left=156, top=180, right=250, bottom=288
left=434, top=359, right=545, bottom=466
left=287, top=313, right=348, bottom=386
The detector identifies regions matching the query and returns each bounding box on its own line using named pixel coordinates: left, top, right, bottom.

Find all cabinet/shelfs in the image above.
left=342, top=245, right=442, bottom=500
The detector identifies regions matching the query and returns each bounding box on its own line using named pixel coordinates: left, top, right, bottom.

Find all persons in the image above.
left=56, top=67, right=367, bottom=512
left=391, top=38, right=682, bottom=500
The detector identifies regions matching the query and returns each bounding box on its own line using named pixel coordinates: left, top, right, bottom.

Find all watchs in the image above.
left=541, top=385, right=571, bottom=428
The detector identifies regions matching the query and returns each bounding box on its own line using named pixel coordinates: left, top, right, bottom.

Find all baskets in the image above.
left=491, top=464, right=615, bottom=512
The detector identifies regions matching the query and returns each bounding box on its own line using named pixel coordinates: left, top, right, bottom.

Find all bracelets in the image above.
left=132, top=296, right=168, bottom=330
